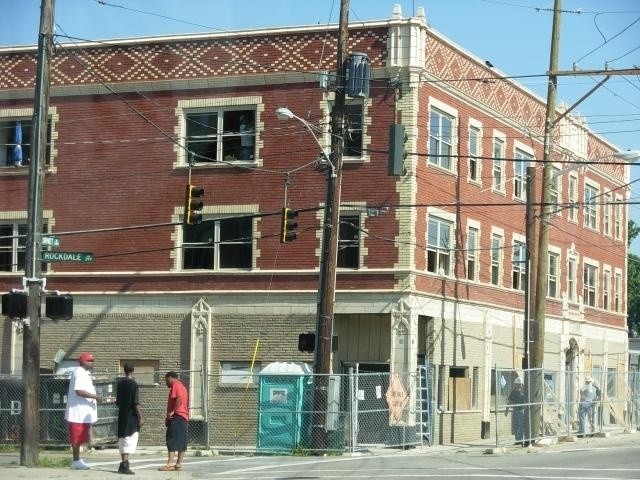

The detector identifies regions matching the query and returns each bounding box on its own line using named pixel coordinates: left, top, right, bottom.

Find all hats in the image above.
left=79, top=353, right=94, bottom=363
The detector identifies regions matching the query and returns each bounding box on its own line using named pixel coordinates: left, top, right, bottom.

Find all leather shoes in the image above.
left=175, top=464, right=184, bottom=471
left=159, top=464, right=175, bottom=472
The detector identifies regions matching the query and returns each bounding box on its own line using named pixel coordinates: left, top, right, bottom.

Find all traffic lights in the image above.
left=1, top=292, right=28, bottom=318
left=279, top=207, right=299, bottom=244
left=387, top=122, right=410, bottom=177
left=184, top=183, right=205, bottom=227
left=297, top=331, right=315, bottom=352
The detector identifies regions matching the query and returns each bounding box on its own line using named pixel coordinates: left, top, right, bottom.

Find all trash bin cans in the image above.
left=0, top=374, right=118, bottom=451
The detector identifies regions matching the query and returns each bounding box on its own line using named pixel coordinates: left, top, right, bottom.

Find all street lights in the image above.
left=274, top=106, right=341, bottom=455
left=523, top=150, right=639, bottom=444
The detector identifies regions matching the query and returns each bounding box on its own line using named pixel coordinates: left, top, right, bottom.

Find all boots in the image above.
left=118, top=461, right=135, bottom=475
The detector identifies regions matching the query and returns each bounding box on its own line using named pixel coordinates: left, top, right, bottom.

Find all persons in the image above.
left=158, top=370, right=191, bottom=471
left=575, top=375, right=596, bottom=437
left=585, top=379, right=601, bottom=431
left=504, top=376, right=525, bottom=443
left=115, top=362, right=143, bottom=475
left=64, top=353, right=104, bottom=470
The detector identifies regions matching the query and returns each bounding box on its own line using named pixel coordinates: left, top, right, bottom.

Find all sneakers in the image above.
left=71, top=461, right=89, bottom=470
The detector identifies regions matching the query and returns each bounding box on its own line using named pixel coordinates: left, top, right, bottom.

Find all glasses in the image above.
left=86, top=359, right=94, bottom=364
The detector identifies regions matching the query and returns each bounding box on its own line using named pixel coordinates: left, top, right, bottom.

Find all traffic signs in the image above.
left=42, top=251, right=94, bottom=263
left=42, top=236, right=60, bottom=246
